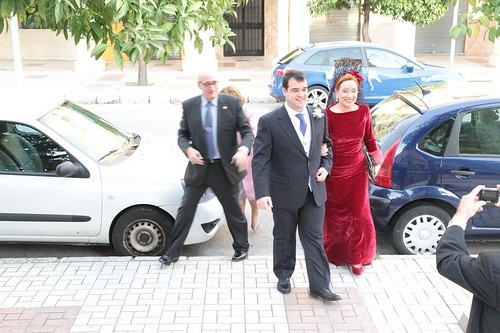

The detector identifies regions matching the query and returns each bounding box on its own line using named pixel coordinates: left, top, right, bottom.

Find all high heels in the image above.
left=351, top=263, right=363, bottom=275
left=251, top=223, right=258, bottom=231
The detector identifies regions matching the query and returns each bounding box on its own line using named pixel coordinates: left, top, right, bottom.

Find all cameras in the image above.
left=479, top=187, right=500, bottom=204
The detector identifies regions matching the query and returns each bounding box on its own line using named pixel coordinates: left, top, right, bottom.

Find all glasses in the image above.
left=199, top=80, right=218, bottom=85
left=338, top=89, right=360, bottom=94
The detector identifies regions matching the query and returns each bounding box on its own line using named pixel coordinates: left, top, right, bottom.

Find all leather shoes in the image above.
left=159, top=255, right=178, bottom=265
left=277, top=279, right=290, bottom=294
left=232, top=252, right=248, bottom=261
left=310, top=289, right=341, bottom=302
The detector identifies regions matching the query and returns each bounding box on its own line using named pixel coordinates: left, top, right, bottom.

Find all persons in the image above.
left=158, top=72, right=259, bottom=265
left=321, top=74, right=383, bottom=275
left=436, top=184, right=500, bottom=333
left=251, top=71, right=342, bottom=302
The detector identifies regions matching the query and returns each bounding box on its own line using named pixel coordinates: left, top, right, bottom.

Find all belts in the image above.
left=206, top=159, right=221, bottom=164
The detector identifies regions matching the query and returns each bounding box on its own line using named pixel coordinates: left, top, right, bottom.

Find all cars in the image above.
left=367, top=82, right=500, bottom=256
left=269, top=40, right=465, bottom=110
left=0, top=93, right=227, bottom=259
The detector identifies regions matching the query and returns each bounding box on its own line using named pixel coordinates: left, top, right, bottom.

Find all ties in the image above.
left=204, top=102, right=215, bottom=160
left=296, top=114, right=307, bottom=136
